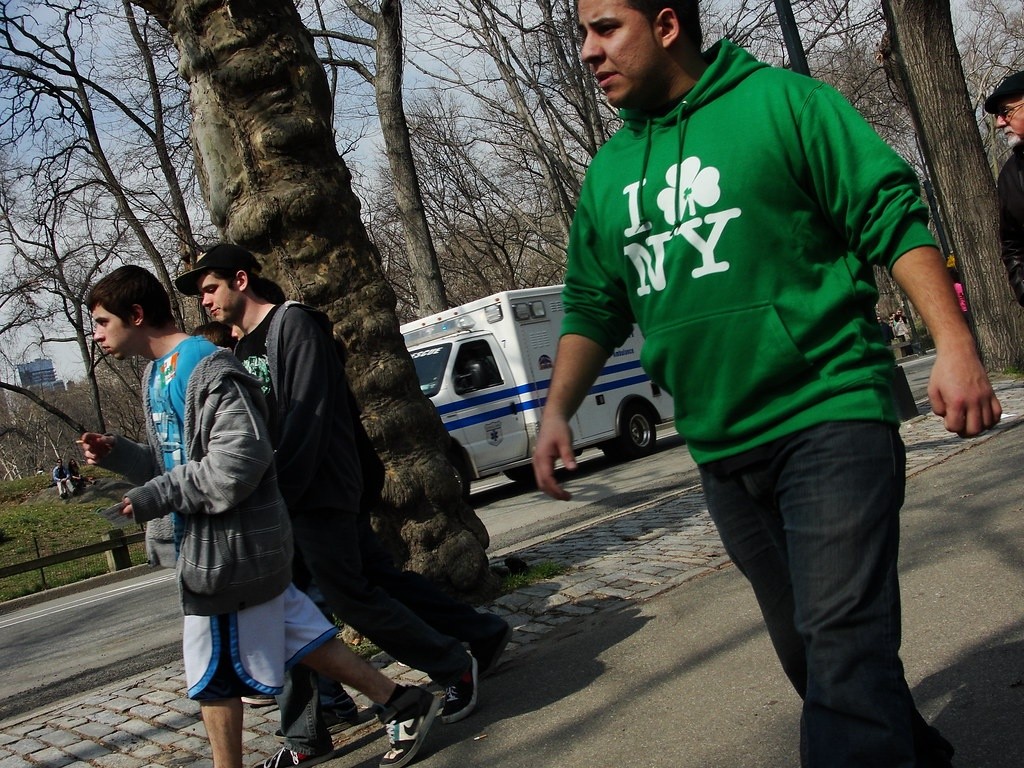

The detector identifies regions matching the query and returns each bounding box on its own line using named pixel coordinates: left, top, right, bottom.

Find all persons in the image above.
left=176, top=244, right=478, bottom=768
left=82, top=263, right=439, bottom=768
left=191, top=279, right=513, bottom=735
left=893, top=315, right=916, bottom=355
left=947, top=265, right=968, bottom=315
left=53, top=458, right=86, bottom=501
left=986, top=70, right=1024, bottom=307
left=533, top=0, right=1004, bottom=768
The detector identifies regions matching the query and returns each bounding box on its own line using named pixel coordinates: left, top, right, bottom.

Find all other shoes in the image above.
left=240, top=693, right=277, bottom=705
left=435, top=640, right=479, bottom=723
left=475, top=612, right=512, bottom=683
left=273, top=693, right=359, bottom=735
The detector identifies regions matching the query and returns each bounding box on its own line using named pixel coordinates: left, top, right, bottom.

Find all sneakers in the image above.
left=254, top=742, right=335, bottom=768
left=373, top=686, right=440, bottom=768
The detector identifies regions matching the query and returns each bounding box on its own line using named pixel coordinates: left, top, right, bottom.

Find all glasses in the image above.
left=995, top=104, right=1024, bottom=116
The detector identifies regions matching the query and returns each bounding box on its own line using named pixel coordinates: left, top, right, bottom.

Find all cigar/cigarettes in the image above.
left=76, top=440, right=85, bottom=444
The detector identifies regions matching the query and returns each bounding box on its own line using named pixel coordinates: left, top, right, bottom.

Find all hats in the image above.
left=985, top=71, right=1024, bottom=112
left=175, top=243, right=262, bottom=296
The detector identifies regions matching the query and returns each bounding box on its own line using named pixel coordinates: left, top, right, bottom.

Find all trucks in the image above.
left=400, top=285, right=674, bottom=484
